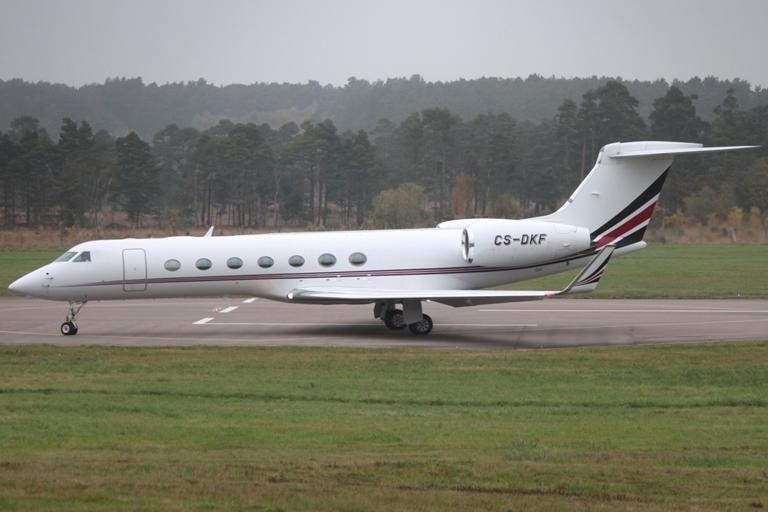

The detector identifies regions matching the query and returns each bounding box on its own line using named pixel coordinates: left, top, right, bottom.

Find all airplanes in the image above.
left=7, top=138, right=764, bottom=336
